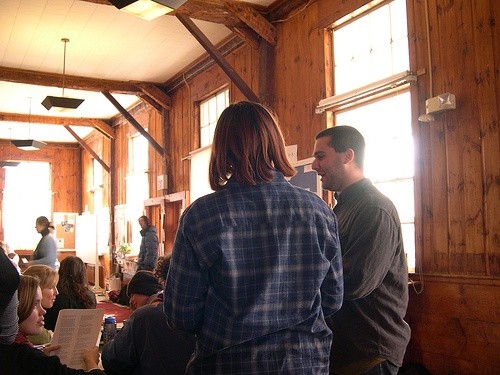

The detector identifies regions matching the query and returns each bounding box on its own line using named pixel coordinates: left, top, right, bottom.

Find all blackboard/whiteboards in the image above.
left=289, top=156, right=328, bottom=201
left=76, top=216, right=101, bottom=269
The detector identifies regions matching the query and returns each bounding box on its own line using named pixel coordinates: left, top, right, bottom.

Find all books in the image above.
left=49, top=309, right=104, bottom=370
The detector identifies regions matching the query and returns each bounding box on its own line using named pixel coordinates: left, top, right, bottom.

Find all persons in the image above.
left=136, top=215, right=158, bottom=271
left=101, top=269, right=163, bottom=375
left=0, top=246, right=20, bottom=345
left=22, top=264, right=59, bottom=344
left=161, top=100, right=343, bottom=375
left=27, top=216, right=57, bottom=270
left=312, top=126, right=411, bottom=375
left=44, top=256, right=97, bottom=331
left=0, top=274, right=104, bottom=375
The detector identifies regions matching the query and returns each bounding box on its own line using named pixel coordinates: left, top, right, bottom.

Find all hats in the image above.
left=126, top=270, right=164, bottom=298
